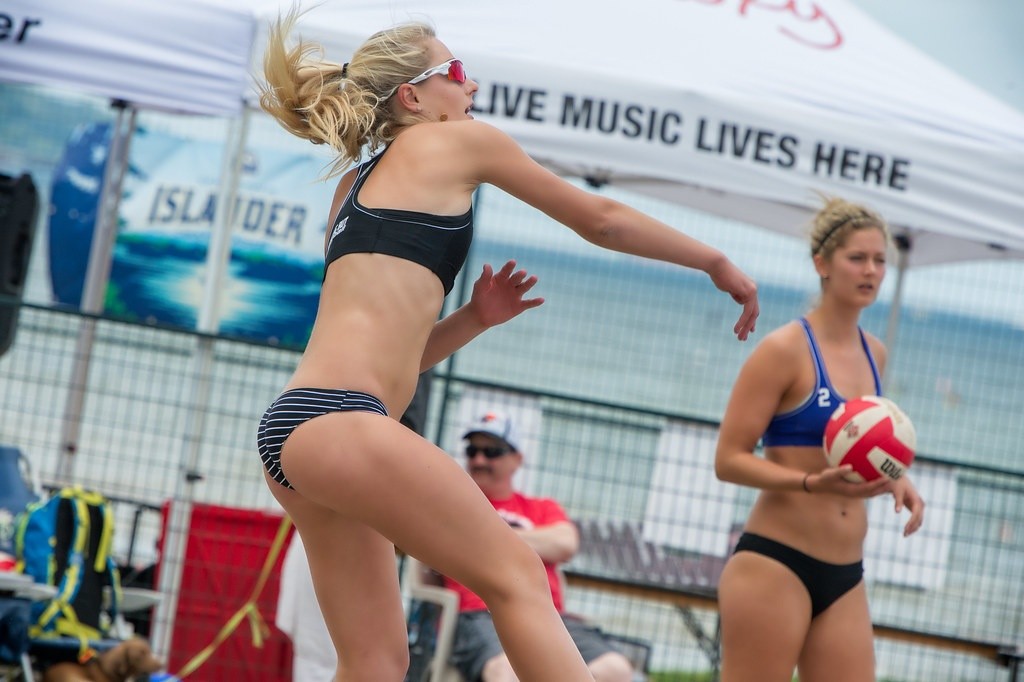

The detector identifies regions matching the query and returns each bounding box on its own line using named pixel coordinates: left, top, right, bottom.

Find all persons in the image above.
left=254, top=2, right=760, bottom=682
left=435, top=410, right=635, bottom=682
left=715, top=194, right=924, bottom=682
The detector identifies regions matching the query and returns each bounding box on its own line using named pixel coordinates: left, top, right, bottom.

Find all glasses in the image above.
left=466, top=444, right=514, bottom=459
left=388, top=58, right=467, bottom=96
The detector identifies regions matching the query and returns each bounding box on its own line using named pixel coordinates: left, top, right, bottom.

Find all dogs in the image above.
left=42, top=637, right=163, bottom=682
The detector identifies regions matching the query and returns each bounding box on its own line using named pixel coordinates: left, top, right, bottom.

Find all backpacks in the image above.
left=11, top=487, right=123, bottom=664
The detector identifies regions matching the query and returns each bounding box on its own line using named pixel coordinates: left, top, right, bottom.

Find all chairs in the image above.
left=401, top=549, right=567, bottom=682
left=0, top=445, right=164, bottom=682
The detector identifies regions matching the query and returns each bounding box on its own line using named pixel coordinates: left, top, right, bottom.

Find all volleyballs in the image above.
left=822, top=394, right=917, bottom=483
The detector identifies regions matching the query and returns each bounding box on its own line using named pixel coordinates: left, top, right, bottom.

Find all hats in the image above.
left=460, top=406, right=527, bottom=456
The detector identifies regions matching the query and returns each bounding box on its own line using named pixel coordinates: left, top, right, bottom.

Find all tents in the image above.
left=1, top=0, right=1024, bottom=682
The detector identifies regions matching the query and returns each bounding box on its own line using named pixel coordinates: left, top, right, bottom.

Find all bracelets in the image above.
left=803, top=473, right=813, bottom=494
